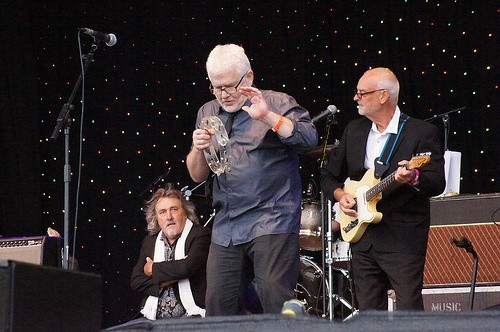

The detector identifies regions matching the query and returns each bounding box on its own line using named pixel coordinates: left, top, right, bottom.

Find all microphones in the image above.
left=82, top=28, right=117, bottom=46
left=312, top=105, right=337, bottom=124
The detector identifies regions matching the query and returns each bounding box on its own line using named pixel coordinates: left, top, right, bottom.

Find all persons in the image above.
left=130, top=183, right=213, bottom=320
left=186, top=44, right=318, bottom=318
left=319, top=67, right=447, bottom=311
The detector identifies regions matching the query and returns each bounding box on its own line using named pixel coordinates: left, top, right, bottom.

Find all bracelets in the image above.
left=408, top=169, right=419, bottom=185
left=272, top=115, right=283, bottom=132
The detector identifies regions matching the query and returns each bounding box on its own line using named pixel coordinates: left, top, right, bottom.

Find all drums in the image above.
left=299, top=197, right=337, bottom=252
left=299, top=254, right=343, bottom=317
left=326, top=240, right=354, bottom=263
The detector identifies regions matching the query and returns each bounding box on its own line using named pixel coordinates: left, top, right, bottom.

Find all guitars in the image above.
left=338, top=151, right=435, bottom=243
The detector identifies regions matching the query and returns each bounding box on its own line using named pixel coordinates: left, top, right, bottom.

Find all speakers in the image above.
left=0, top=259, right=102, bottom=332
left=0, top=236, right=64, bottom=268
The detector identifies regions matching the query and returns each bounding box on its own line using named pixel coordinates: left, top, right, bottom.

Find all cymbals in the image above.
left=309, top=143, right=339, bottom=155
left=198, top=114, right=233, bottom=178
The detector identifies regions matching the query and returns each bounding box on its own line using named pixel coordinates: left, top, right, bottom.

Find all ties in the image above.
left=205, top=110, right=244, bottom=195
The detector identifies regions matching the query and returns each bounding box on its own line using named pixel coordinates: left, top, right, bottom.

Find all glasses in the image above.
left=355, top=89, right=384, bottom=99
left=209, top=71, right=248, bottom=94
